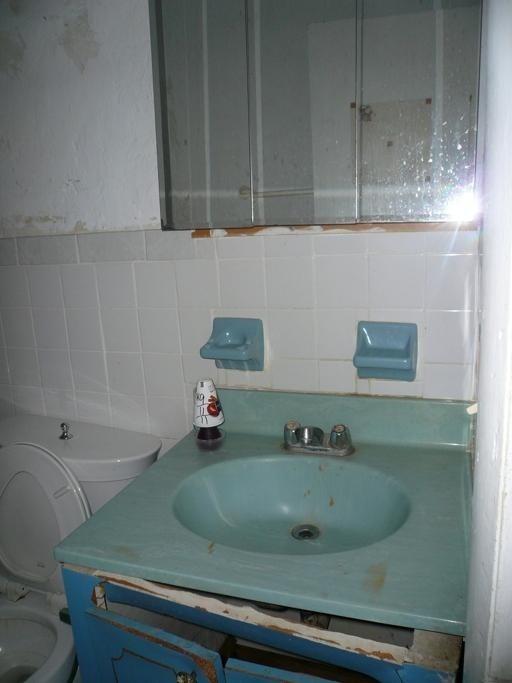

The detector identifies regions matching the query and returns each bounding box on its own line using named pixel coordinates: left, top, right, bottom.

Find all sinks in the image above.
left=170, top=453, right=413, bottom=558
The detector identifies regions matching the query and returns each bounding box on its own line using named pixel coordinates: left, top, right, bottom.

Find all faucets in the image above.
left=295, top=424, right=325, bottom=448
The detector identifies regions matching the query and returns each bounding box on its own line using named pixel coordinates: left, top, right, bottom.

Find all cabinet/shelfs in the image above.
left=61, top=562, right=465, bottom=682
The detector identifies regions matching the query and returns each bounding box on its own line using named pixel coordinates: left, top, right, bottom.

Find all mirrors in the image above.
left=149, top=0, right=487, bottom=231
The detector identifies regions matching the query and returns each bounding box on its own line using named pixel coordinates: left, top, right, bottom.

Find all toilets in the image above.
left=1, top=411, right=163, bottom=683
